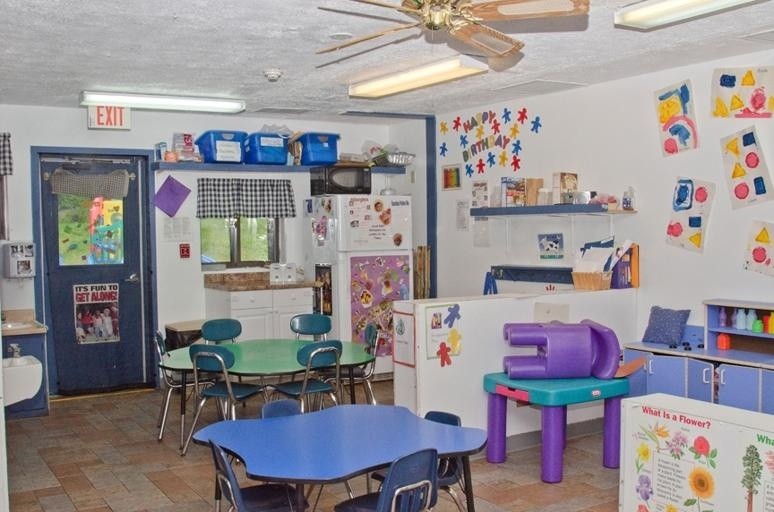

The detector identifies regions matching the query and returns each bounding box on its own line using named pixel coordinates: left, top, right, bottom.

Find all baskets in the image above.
left=571, top=271, right=613, bottom=291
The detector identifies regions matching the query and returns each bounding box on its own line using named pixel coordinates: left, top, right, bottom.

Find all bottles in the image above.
left=718, top=307, right=774, bottom=334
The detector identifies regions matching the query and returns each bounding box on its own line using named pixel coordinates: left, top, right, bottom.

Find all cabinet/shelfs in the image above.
left=622, top=341, right=688, bottom=399
left=204, top=280, right=314, bottom=341
left=701, top=298, right=774, bottom=356
left=2, top=333, right=50, bottom=420
left=688, top=345, right=774, bottom=413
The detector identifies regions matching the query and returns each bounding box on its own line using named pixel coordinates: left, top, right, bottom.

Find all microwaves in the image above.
left=310, top=165, right=372, bottom=195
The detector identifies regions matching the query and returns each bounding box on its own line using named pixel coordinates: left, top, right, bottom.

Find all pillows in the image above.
left=641, top=306, right=691, bottom=345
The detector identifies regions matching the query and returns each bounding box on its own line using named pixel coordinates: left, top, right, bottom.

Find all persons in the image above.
left=393, top=233, right=401, bottom=246
left=78, top=305, right=118, bottom=337
left=374, top=201, right=382, bottom=211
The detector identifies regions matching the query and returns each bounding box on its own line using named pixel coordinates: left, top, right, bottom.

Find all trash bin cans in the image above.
left=165, top=318, right=216, bottom=393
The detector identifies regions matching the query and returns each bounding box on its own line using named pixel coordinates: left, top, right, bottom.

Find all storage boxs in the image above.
left=294, top=131, right=339, bottom=169
left=245, top=132, right=286, bottom=165
left=194, top=130, right=245, bottom=161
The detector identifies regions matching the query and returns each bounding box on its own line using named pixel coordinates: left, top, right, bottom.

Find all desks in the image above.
left=153, top=337, right=375, bottom=450
left=484, top=365, right=629, bottom=483
left=189, top=404, right=488, bottom=512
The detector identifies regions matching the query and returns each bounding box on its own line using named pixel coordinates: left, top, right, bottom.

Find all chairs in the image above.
left=315, top=320, right=380, bottom=411
left=206, top=440, right=307, bottom=511
left=260, top=398, right=354, bottom=512
left=270, top=313, right=331, bottom=398
left=149, top=329, right=213, bottom=441
left=371, top=411, right=466, bottom=512
left=334, top=448, right=436, bottom=512
left=201, top=318, right=250, bottom=409
left=180, top=340, right=256, bottom=455
left=260, top=339, right=342, bottom=414
left=499, top=317, right=619, bottom=379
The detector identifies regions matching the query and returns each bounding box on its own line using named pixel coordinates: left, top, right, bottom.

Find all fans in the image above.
left=315, top=0, right=589, bottom=58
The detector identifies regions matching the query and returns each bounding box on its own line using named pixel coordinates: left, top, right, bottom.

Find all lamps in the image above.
left=79, top=91, right=246, bottom=117
left=612, top=0, right=759, bottom=34
left=348, top=57, right=489, bottom=98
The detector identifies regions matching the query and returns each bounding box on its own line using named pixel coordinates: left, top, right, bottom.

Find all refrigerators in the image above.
left=302, top=193, right=415, bottom=382
